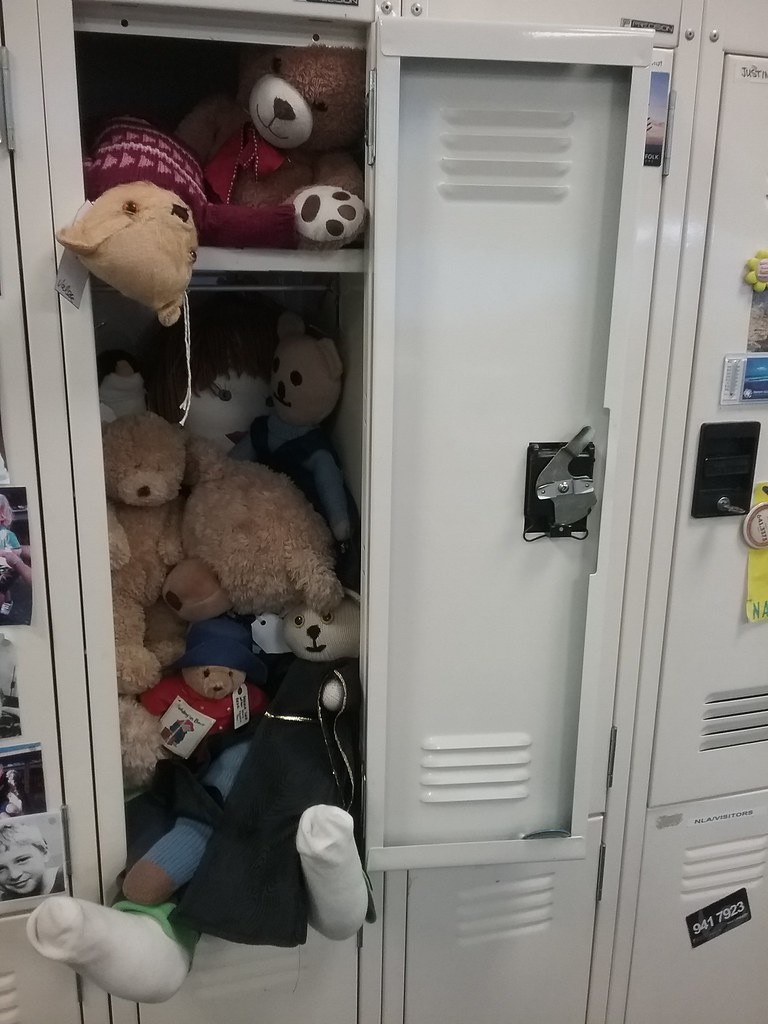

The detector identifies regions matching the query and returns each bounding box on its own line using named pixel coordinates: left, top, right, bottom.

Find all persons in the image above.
left=0, top=823, right=66, bottom=901
left=0, top=495, right=32, bottom=616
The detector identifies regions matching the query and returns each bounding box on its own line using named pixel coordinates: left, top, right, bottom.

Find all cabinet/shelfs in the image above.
left=37, top=0, right=654, bottom=1023
left=382, top=0, right=703, bottom=1023
left=605, top=0, right=768, bottom=1024
left=0, top=0, right=82, bottom=1024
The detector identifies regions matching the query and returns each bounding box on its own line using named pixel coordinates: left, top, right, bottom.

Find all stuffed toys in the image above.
left=26, top=45, right=379, bottom=1004
